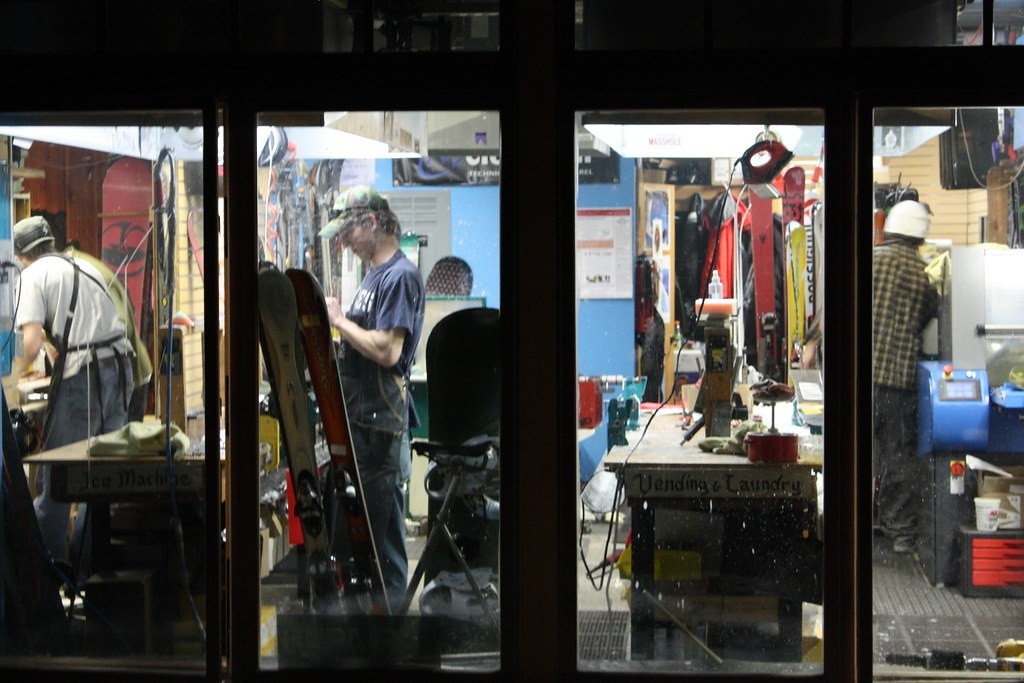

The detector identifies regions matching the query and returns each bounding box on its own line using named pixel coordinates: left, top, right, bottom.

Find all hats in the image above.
left=883, top=199, right=931, bottom=239
left=12, top=215, right=55, bottom=254
left=317, top=185, right=390, bottom=239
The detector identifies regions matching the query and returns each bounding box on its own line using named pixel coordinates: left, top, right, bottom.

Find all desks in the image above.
left=17, top=427, right=273, bottom=658
left=603, top=400, right=823, bottom=665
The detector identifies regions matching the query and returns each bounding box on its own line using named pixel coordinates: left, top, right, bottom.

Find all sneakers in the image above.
left=893, top=533, right=920, bottom=553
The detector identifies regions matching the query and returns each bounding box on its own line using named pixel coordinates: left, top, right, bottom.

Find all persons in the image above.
left=871, top=197, right=945, bottom=554
left=321, top=185, right=424, bottom=615
left=3, top=215, right=138, bottom=576
left=41, top=216, right=153, bottom=424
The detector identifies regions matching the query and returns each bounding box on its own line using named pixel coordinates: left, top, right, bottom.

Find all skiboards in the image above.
left=286, top=266, right=395, bottom=615
left=676, top=164, right=818, bottom=385
left=16, top=138, right=352, bottom=453
left=257, top=268, right=345, bottom=617
